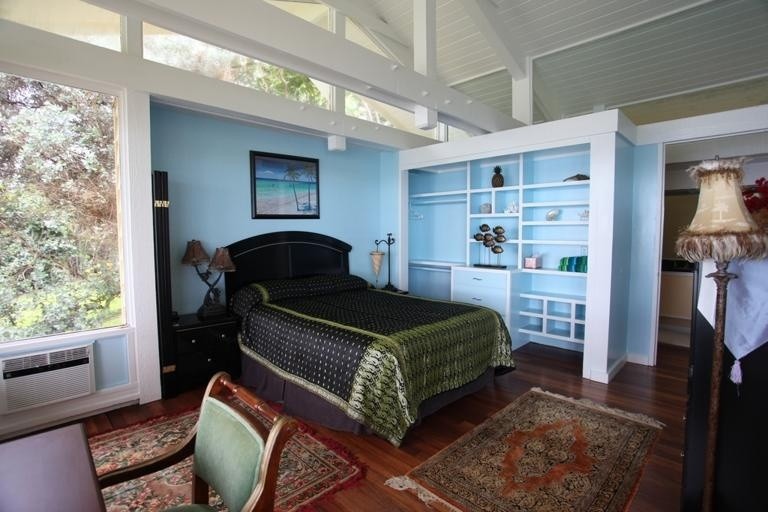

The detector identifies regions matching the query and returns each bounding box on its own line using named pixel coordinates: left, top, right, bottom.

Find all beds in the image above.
left=225, top=231, right=517, bottom=449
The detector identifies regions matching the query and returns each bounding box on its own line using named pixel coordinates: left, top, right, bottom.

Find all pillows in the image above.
left=233, top=272, right=374, bottom=319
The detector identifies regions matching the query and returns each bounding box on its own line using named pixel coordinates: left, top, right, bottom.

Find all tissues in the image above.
left=525, top=252, right=540, bottom=269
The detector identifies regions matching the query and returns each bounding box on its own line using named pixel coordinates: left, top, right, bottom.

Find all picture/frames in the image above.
left=251, top=149, right=320, bottom=222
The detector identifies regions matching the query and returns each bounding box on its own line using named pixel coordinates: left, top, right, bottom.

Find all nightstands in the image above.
left=161, top=311, right=242, bottom=398
left=395, top=289, right=409, bottom=296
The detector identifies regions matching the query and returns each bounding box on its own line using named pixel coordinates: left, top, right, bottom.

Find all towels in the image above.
left=558, top=256, right=588, bottom=273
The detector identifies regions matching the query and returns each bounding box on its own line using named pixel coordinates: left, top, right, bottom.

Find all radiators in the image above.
left=0, top=341, right=97, bottom=416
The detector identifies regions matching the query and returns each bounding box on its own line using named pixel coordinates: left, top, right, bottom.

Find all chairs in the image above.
left=97, top=371, right=298, bottom=512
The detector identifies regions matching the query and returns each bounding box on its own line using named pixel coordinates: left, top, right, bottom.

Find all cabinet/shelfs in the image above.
left=521, top=288, right=584, bottom=353
left=449, top=268, right=532, bottom=350
left=408, top=168, right=468, bottom=265
left=522, top=152, right=591, bottom=271
left=469, top=154, right=521, bottom=268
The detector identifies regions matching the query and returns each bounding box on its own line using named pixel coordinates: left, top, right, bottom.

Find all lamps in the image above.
left=369, top=233, right=398, bottom=292
left=180, top=237, right=237, bottom=317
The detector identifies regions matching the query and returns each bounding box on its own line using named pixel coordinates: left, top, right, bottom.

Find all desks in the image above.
left=1, top=421, right=110, bottom=512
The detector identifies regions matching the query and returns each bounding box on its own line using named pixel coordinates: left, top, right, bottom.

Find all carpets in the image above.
left=383, top=387, right=668, bottom=509
left=86, top=400, right=370, bottom=512
